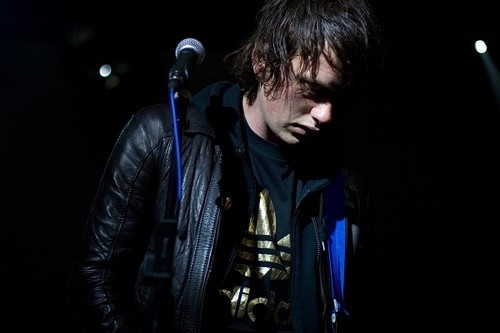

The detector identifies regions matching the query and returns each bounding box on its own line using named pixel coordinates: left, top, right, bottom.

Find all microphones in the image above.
left=168, top=36, right=206, bottom=87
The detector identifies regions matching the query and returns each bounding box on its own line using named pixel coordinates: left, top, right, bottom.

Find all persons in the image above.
left=78, top=1, right=381, bottom=333
